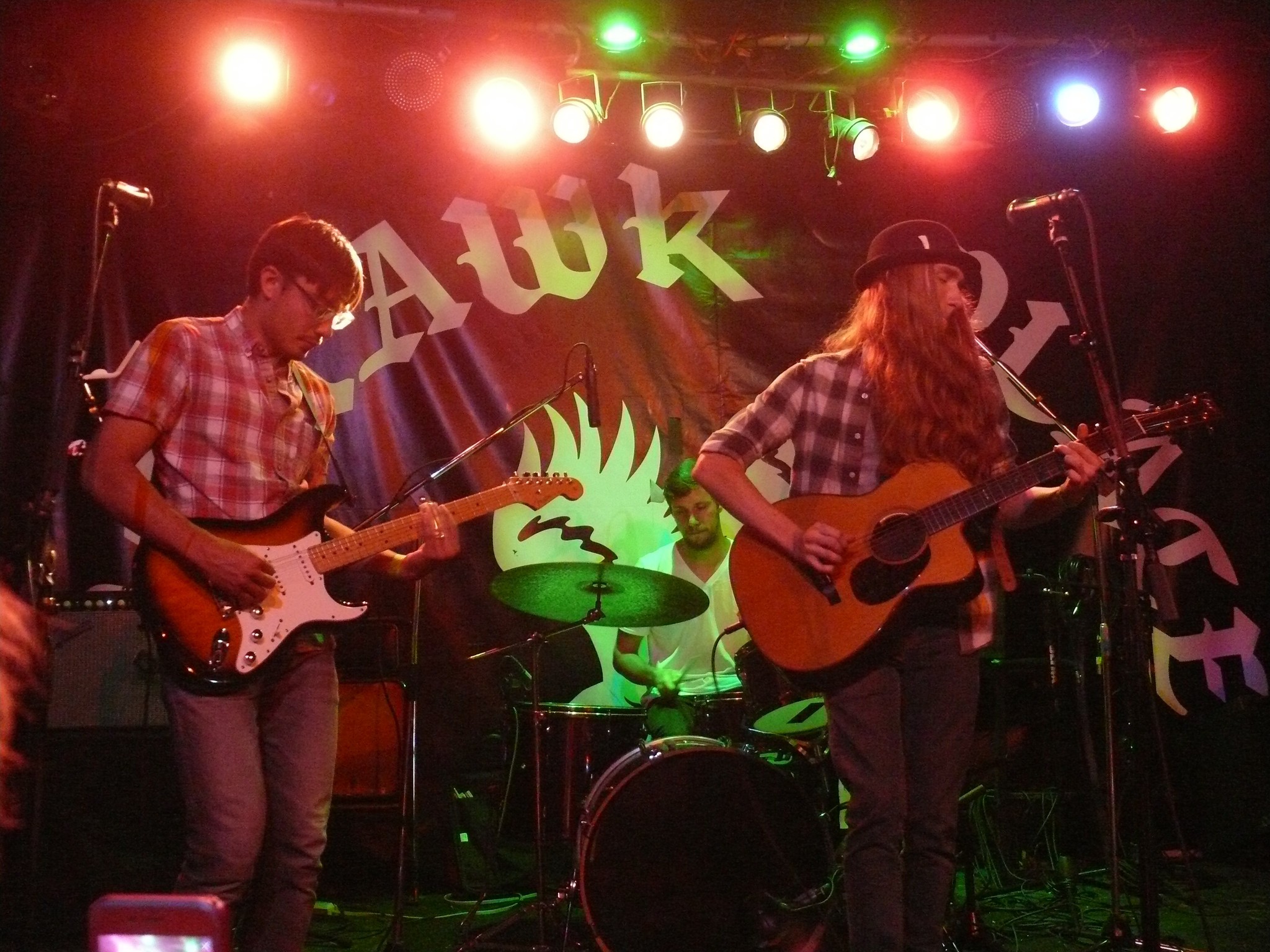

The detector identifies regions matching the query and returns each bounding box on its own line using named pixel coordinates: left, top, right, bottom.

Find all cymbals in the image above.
left=491, top=561, right=710, bottom=627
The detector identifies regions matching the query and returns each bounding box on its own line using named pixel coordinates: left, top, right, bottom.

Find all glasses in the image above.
left=286, top=273, right=355, bottom=330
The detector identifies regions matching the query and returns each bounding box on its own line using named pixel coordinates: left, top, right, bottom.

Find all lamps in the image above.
left=733, top=84, right=795, bottom=157
left=809, top=90, right=881, bottom=179
left=883, top=77, right=960, bottom=146
left=551, top=73, right=619, bottom=145
left=640, top=80, right=687, bottom=150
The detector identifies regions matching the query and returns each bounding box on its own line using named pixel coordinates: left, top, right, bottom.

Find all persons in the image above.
left=79, top=219, right=461, bottom=951
left=612, top=458, right=752, bottom=742
left=691, top=221, right=1106, bottom=952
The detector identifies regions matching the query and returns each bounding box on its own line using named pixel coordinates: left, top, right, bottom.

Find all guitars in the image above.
left=131, top=472, right=584, bottom=696
left=729, top=391, right=1229, bottom=674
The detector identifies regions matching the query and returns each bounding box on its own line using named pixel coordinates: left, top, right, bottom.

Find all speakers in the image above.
left=50, top=609, right=176, bottom=728
left=331, top=620, right=410, bottom=799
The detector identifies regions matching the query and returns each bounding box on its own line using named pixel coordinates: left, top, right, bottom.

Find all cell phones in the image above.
left=89, top=894, right=232, bottom=952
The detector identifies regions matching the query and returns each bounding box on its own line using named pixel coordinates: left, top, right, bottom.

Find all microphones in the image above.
left=583, top=345, right=603, bottom=432
left=1002, top=183, right=1078, bottom=227
left=102, top=175, right=156, bottom=215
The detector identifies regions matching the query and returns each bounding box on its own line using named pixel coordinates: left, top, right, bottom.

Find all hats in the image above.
left=854, top=219, right=981, bottom=292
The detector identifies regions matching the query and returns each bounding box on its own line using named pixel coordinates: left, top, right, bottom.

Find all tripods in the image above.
left=463, top=647, right=588, bottom=951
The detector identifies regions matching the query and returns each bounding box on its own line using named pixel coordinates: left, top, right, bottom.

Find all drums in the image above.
left=734, top=640, right=829, bottom=736
left=693, top=691, right=752, bottom=746
left=492, top=699, right=648, bottom=850
left=578, top=736, right=835, bottom=952
